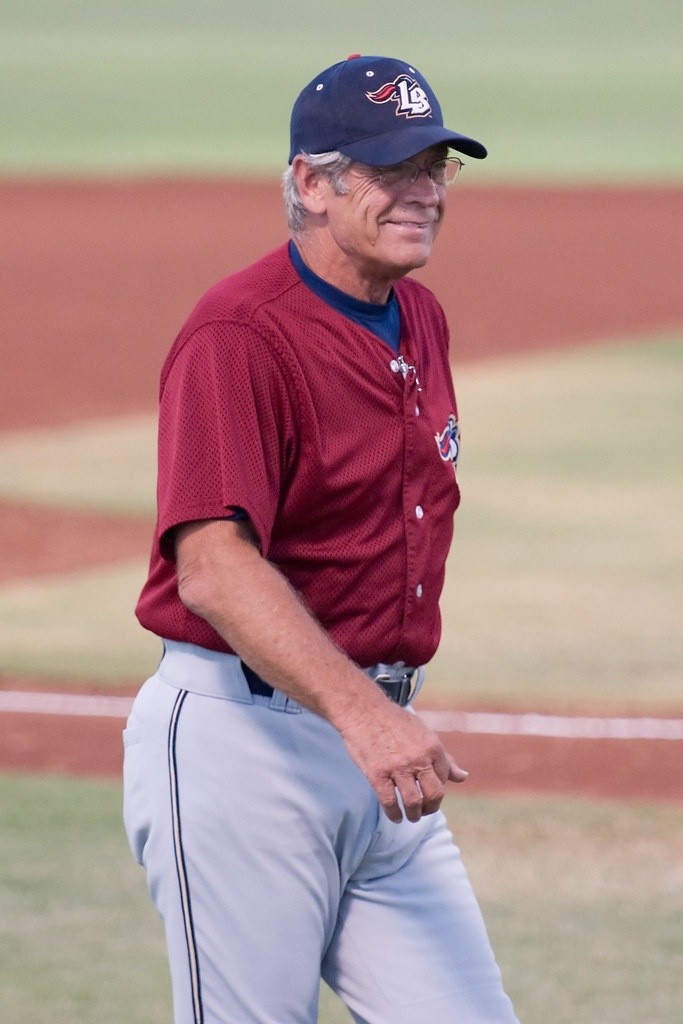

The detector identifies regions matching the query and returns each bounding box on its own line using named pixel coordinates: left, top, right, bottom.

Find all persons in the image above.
left=122, top=53, right=519, bottom=1024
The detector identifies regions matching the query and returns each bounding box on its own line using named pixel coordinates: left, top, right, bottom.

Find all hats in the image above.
left=289, top=53, right=487, bottom=169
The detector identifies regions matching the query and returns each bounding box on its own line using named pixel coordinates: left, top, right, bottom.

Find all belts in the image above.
left=242, top=659, right=412, bottom=714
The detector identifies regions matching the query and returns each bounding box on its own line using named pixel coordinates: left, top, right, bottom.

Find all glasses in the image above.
left=328, top=156, right=465, bottom=193
